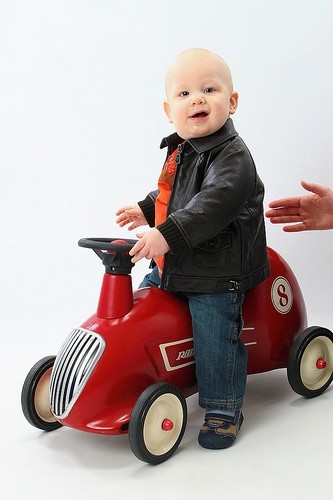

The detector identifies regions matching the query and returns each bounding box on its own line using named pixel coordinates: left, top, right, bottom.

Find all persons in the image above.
left=265, top=178, right=333, bottom=241
left=117, top=47, right=270, bottom=452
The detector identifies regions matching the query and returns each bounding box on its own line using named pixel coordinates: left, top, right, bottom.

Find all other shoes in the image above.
left=198, top=410, right=244, bottom=449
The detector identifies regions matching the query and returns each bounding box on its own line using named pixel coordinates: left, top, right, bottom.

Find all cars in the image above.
left=21, top=236, right=333, bottom=465
